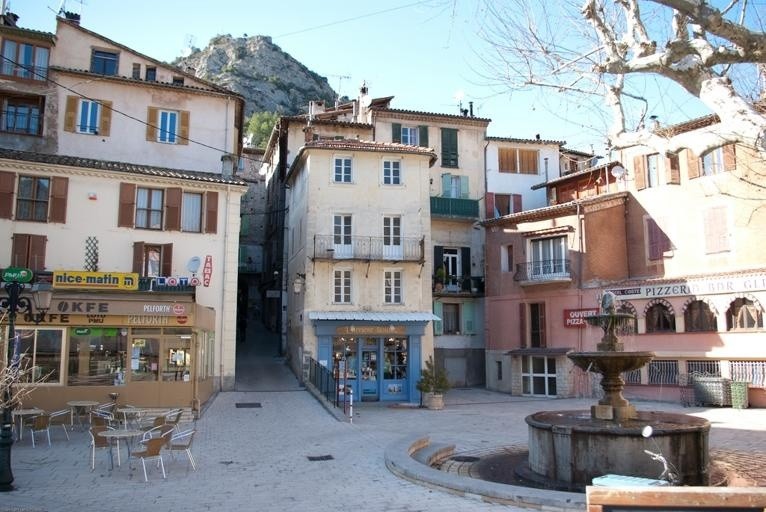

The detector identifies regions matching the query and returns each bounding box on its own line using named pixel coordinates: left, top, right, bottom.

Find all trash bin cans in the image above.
left=727, top=381, right=752, bottom=409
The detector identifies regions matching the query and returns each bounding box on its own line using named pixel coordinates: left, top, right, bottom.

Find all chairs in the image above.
left=22, top=408, right=197, bottom=482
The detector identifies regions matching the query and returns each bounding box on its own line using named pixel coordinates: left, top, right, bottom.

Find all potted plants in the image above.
left=416, top=356, right=450, bottom=410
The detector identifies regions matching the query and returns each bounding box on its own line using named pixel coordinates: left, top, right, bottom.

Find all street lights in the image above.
left=0, top=280, right=56, bottom=492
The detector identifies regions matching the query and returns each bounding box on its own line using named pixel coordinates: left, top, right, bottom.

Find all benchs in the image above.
left=585, top=485, right=766, bottom=512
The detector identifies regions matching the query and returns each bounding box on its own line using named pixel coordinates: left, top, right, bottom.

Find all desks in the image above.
left=12, top=410, right=45, bottom=441
left=65, top=400, right=100, bottom=432
